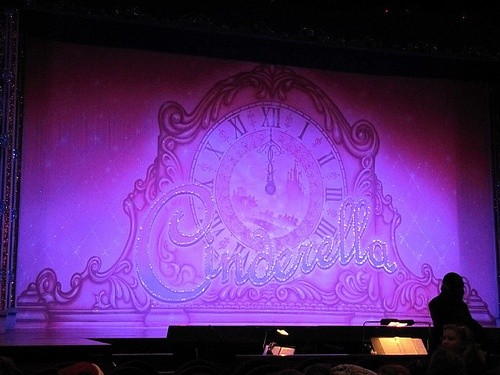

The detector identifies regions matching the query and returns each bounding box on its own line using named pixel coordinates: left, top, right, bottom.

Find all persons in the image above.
left=428, top=272, right=486, bottom=350
left=0, top=324, right=500, bottom=375
